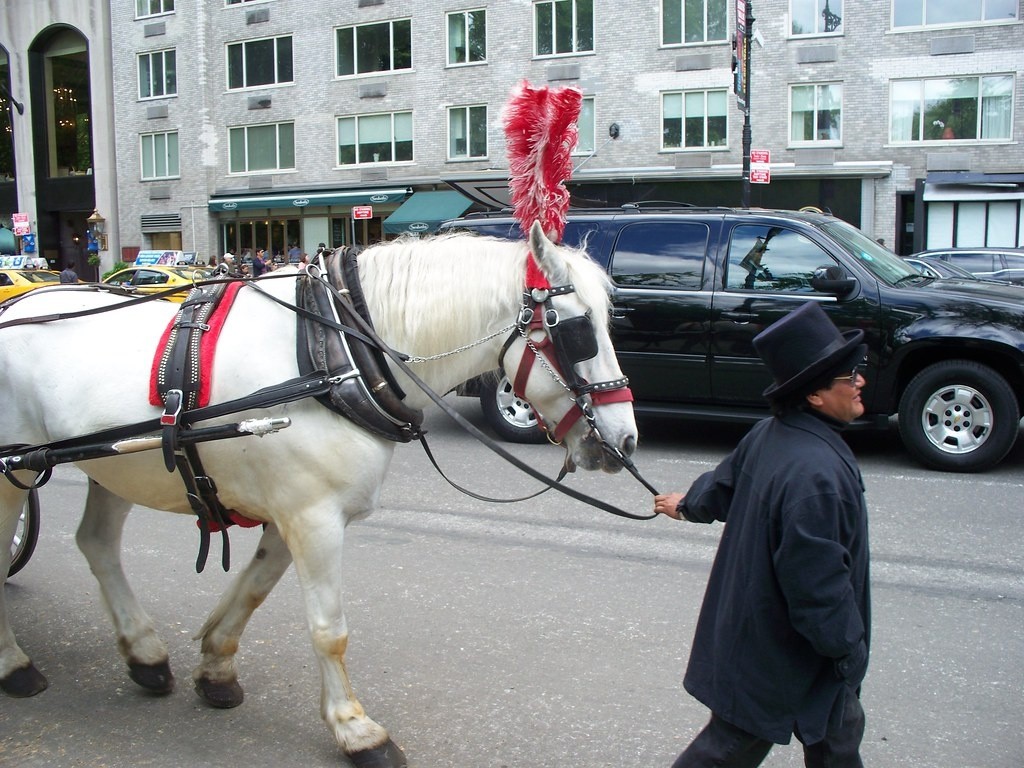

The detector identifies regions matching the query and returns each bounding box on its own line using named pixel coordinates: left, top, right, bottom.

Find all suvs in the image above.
left=424, top=209, right=1024, bottom=474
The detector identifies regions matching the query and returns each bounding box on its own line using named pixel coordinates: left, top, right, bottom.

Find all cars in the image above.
left=101, top=265, right=217, bottom=304
left=0, top=255, right=90, bottom=307
left=903, top=241, right=1024, bottom=285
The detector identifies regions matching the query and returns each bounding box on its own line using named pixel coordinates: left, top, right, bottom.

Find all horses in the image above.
left=1, top=220, right=639, bottom=768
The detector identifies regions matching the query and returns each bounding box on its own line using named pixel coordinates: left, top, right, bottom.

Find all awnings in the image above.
left=208, top=184, right=413, bottom=212
left=383, top=190, right=474, bottom=234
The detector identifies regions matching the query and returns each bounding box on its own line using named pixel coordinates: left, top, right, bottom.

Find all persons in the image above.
left=877, top=239, right=885, bottom=246
left=177, top=240, right=326, bottom=277
left=652, top=301, right=872, bottom=768
left=60, top=262, right=78, bottom=283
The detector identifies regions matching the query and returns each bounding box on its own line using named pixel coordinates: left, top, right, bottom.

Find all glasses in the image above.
left=828, top=365, right=861, bottom=387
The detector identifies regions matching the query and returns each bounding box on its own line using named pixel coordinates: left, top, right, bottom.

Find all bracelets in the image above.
left=679, top=511, right=687, bottom=521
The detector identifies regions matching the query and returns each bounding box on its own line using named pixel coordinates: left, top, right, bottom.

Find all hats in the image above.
left=753, top=298, right=867, bottom=405
